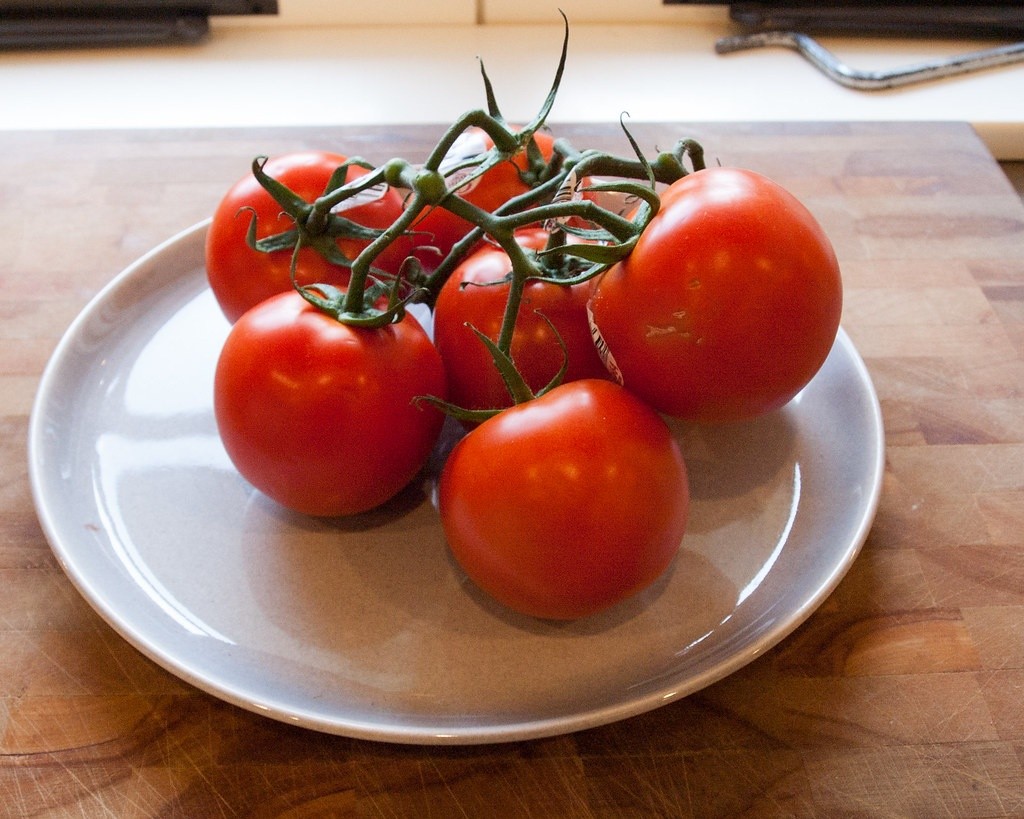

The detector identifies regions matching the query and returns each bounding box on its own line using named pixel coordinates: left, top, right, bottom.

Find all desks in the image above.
left=0, top=120, right=1023, bottom=819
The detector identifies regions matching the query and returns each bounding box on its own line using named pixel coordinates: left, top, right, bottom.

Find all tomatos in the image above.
left=203, top=125, right=845, bottom=619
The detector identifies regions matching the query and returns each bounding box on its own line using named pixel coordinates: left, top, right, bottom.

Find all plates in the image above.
left=25, top=216, right=885, bottom=746
